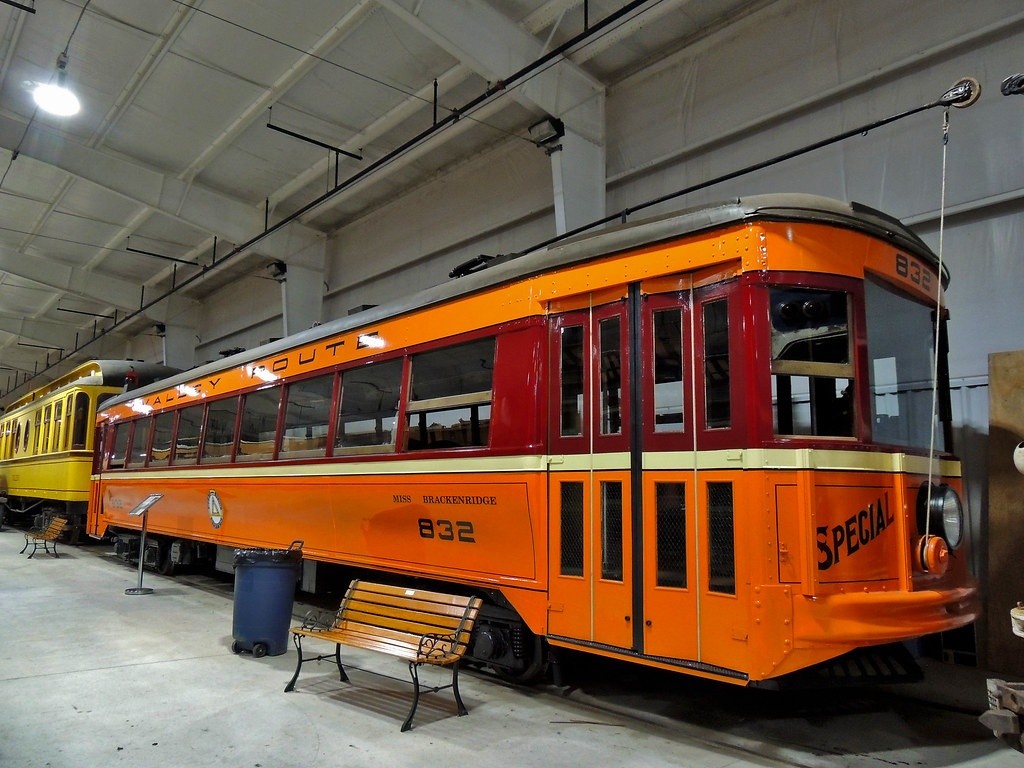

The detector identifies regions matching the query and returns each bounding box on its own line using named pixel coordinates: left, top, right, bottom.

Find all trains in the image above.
left=0, top=360, right=187, bottom=545
left=87, top=193, right=985, bottom=687
left=379, top=462, right=385, bottom=464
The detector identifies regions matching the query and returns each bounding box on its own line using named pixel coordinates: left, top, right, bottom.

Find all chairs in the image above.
left=148, top=419, right=491, bottom=462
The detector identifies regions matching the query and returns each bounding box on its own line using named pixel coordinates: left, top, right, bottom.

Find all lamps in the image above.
left=33, top=54, right=83, bottom=119
left=266, top=261, right=288, bottom=278
left=527, top=112, right=566, bottom=147
left=151, top=324, right=166, bottom=334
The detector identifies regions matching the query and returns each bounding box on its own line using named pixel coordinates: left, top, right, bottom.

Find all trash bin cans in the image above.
left=232, top=540, right=305, bottom=658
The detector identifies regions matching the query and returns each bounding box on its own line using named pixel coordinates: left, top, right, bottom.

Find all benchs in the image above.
left=19, top=516, right=70, bottom=560
left=285, top=580, right=489, bottom=734
